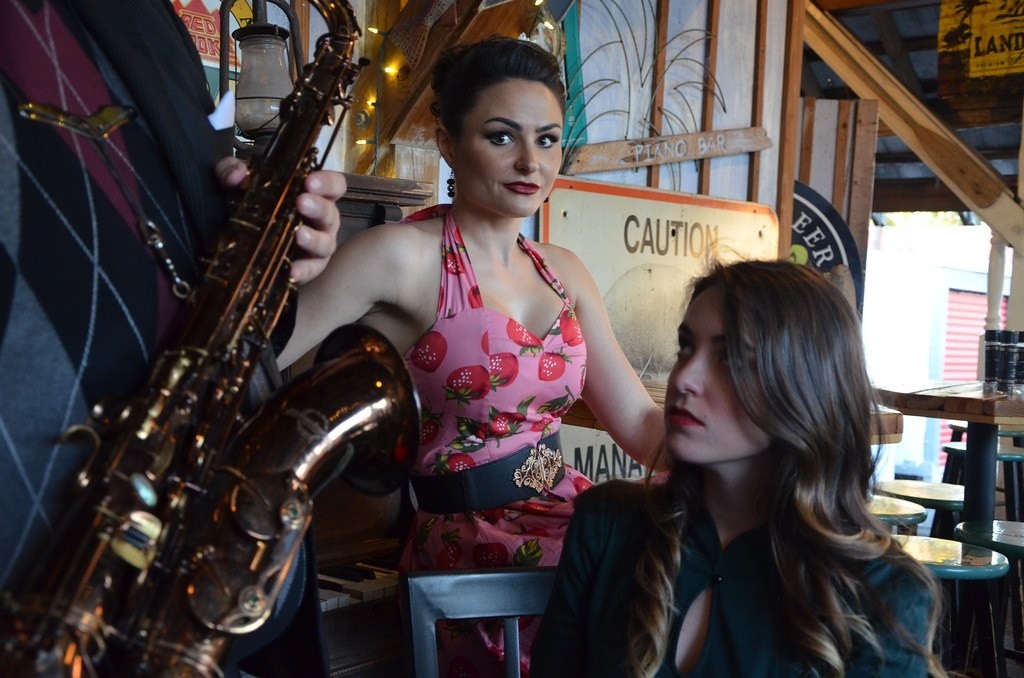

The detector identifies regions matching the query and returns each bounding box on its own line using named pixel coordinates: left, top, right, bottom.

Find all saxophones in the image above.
left=1, top=1, right=425, bottom=678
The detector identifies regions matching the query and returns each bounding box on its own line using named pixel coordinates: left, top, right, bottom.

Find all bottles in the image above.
left=984, top=329, right=1003, bottom=383
left=997, top=330, right=1024, bottom=393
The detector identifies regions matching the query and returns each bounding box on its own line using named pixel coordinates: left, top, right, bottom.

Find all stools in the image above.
left=948, top=422, right=1024, bottom=522
left=866, top=494, right=927, bottom=535
left=953, top=520, right=1024, bottom=678
left=875, top=480, right=966, bottom=678
left=929, top=441, right=1024, bottom=539
left=889, top=533, right=1010, bottom=678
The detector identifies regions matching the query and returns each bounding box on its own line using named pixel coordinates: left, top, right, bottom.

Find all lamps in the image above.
left=219, top=0, right=306, bottom=163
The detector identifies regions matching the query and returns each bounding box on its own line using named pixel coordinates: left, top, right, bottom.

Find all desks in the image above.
left=556, top=382, right=904, bottom=444
left=871, top=380, right=1024, bottom=678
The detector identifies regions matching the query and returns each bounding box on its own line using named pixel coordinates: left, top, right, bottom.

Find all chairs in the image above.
left=400, top=566, right=560, bottom=678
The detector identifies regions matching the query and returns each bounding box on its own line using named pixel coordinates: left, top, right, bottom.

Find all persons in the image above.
left=529, top=259, right=949, bottom=678
left=276, top=34, right=668, bottom=678
left=0, top=0, right=349, bottom=678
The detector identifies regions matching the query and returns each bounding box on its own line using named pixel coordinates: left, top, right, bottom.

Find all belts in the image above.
left=411, top=431, right=566, bottom=514
